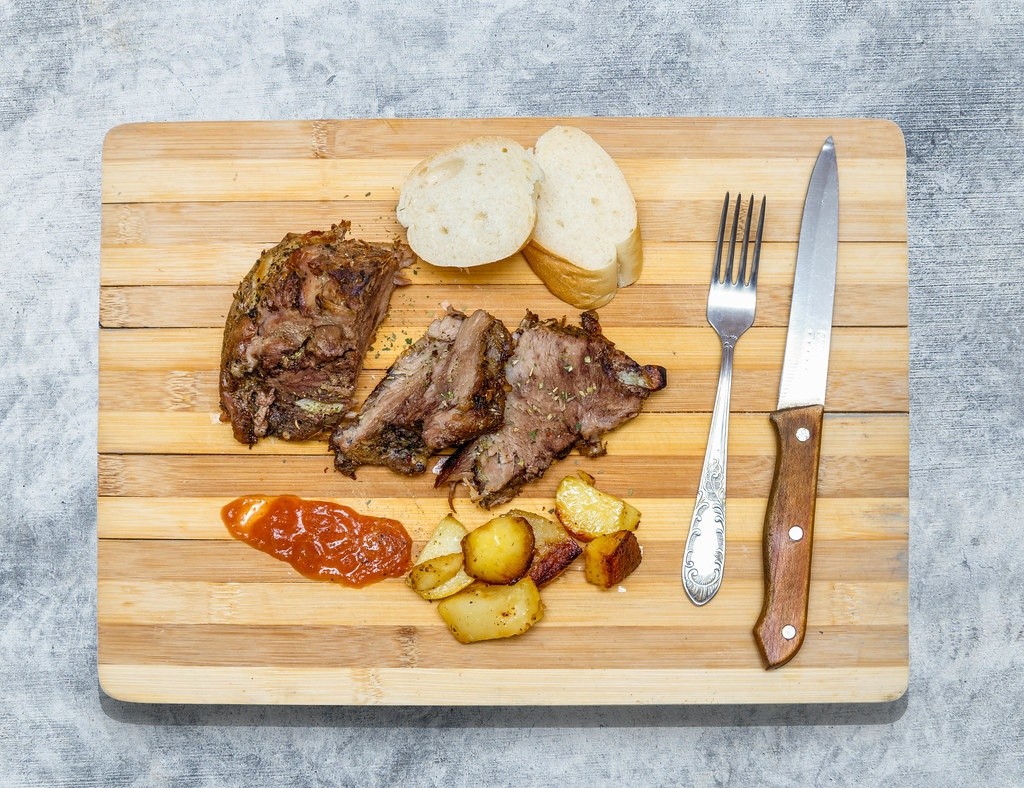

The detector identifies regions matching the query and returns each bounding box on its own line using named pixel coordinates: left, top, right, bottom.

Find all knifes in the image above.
left=751, top=136, right=839, bottom=668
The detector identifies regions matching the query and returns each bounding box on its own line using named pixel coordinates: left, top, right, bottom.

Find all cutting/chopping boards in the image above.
left=91, top=116, right=913, bottom=707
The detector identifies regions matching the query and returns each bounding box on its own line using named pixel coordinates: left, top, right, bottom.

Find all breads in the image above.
left=394, top=133, right=544, bottom=268
left=520, top=125, right=643, bottom=310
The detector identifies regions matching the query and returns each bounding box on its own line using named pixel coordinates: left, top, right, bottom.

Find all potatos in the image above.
left=405, top=471, right=645, bottom=643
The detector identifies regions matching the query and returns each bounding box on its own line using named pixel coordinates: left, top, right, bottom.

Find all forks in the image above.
left=679, top=192, right=768, bottom=606
